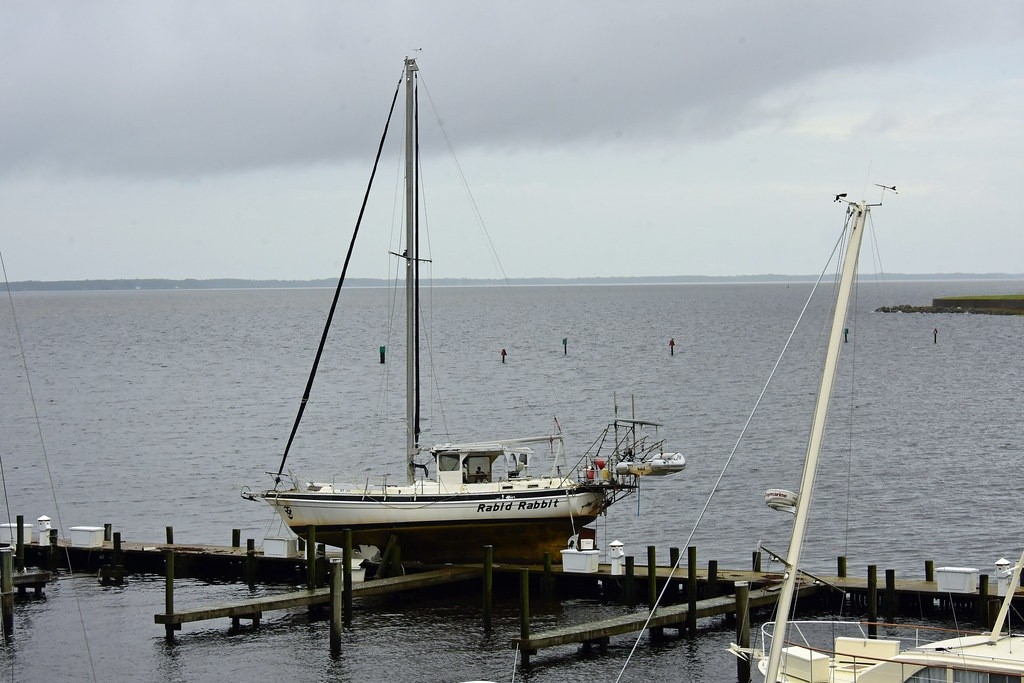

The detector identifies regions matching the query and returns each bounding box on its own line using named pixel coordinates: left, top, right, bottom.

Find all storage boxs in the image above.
left=341, top=559, right=366, bottom=582
left=263, top=536, right=298, bottom=558
left=834, top=636, right=901, bottom=664
left=0, top=523, right=33, bottom=544
left=69, top=526, right=105, bottom=547
left=780, top=646, right=830, bottom=683
left=935, top=566, right=978, bottom=593
left=560, top=548, right=601, bottom=573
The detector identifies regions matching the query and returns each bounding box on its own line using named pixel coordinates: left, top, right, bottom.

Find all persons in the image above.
left=476, top=466, right=488, bottom=484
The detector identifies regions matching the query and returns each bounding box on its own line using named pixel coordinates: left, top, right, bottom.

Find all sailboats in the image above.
left=241, top=51, right=686, bottom=565
left=727, top=547, right=1024, bottom=683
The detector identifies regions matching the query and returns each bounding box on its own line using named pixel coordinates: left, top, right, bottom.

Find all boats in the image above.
left=649, top=453, right=685, bottom=472
